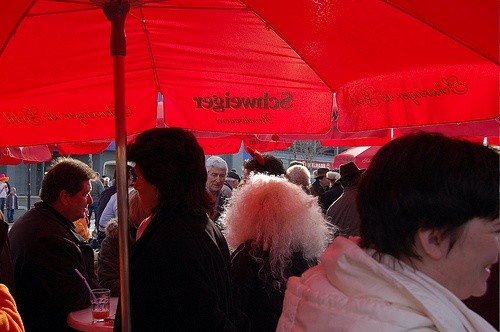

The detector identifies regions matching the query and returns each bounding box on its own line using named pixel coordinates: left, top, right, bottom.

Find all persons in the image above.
left=278, top=129, right=500, bottom=332
left=205, top=147, right=367, bottom=332
left=0, top=209, right=25, bottom=332
left=71, top=162, right=154, bottom=301
left=112, top=126, right=236, bottom=332
left=8, top=157, right=99, bottom=332
left=0, top=174, right=10, bottom=213
left=6, top=187, right=19, bottom=223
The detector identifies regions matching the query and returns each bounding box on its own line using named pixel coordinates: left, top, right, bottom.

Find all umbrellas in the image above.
left=0, top=95, right=500, bottom=165
left=333, top=146, right=383, bottom=170
left=0, top=0, right=500, bottom=332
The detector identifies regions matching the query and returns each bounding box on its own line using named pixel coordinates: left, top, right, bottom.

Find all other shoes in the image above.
left=9, top=221, right=14, bottom=223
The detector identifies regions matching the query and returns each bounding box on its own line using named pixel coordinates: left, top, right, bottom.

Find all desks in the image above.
left=67, top=296, right=118, bottom=332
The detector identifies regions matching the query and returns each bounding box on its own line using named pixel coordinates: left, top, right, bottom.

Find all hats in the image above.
left=335, top=161, right=366, bottom=183
left=227, top=172, right=240, bottom=182
left=0, top=174, right=9, bottom=181
left=314, top=168, right=329, bottom=179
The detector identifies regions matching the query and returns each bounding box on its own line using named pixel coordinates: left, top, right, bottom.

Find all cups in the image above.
left=89, top=288, right=111, bottom=322
